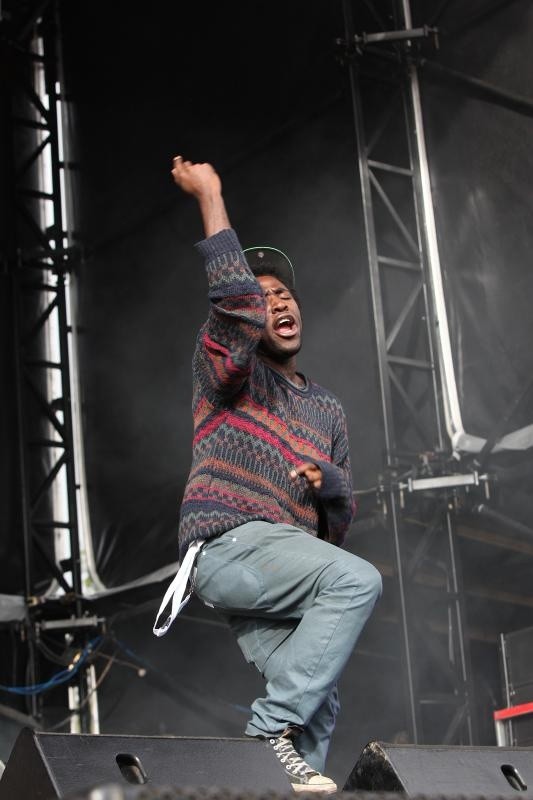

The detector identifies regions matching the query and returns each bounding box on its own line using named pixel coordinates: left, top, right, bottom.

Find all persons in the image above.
left=169, top=156, right=383, bottom=792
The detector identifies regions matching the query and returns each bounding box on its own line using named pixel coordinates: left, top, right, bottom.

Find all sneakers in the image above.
left=246, top=726, right=337, bottom=795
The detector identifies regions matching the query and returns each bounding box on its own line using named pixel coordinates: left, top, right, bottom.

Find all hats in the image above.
left=243, top=242, right=295, bottom=291
